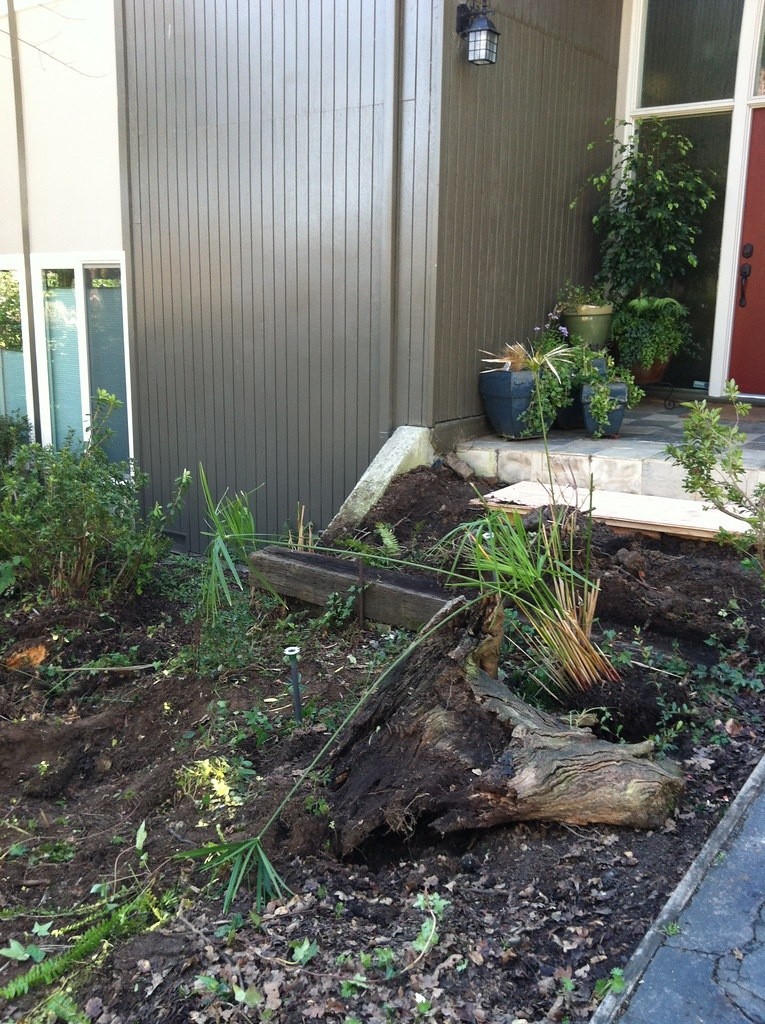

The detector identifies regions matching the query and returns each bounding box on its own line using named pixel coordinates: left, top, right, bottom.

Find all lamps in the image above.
left=456, top=0, right=501, bottom=66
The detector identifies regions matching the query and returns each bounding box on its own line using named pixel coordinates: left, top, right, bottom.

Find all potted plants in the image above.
left=614, top=305, right=690, bottom=384
left=478, top=329, right=573, bottom=439
left=578, top=364, right=646, bottom=437
left=556, top=279, right=614, bottom=346
left=555, top=342, right=615, bottom=428
